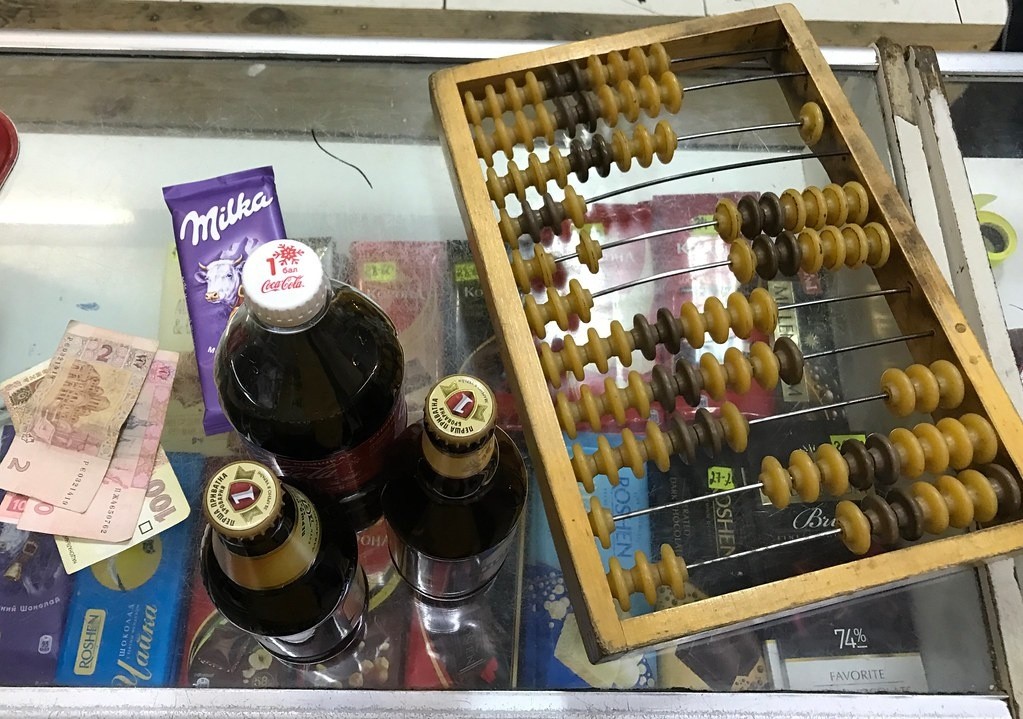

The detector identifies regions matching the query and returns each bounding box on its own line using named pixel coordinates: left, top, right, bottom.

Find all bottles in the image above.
left=215, top=242, right=406, bottom=532
left=380, top=374, right=527, bottom=601
left=198, top=459, right=369, bottom=670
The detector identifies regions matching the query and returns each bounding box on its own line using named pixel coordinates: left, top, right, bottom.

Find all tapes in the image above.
left=977, top=211, right=1016, bottom=263
